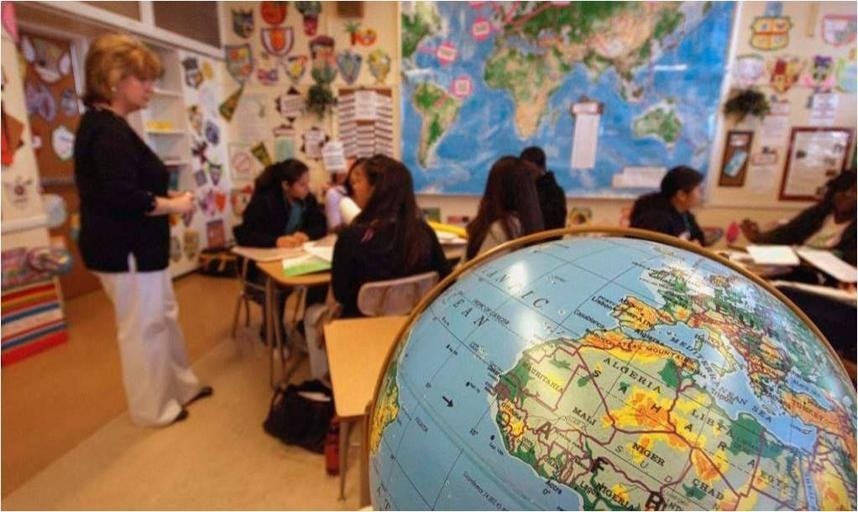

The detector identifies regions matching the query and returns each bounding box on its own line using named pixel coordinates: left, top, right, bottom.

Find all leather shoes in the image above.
left=175, top=386, right=213, bottom=422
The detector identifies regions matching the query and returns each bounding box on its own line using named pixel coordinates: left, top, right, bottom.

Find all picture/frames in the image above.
left=719, top=129, right=755, bottom=186
left=777, top=125, right=854, bottom=201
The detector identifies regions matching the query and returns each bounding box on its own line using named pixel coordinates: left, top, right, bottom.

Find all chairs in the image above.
left=231, top=255, right=281, bottom=340
left=357, top=270, right=441, bottom=316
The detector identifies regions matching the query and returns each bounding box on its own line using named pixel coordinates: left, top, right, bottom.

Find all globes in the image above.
left=367, top=224, right=858, bottom=512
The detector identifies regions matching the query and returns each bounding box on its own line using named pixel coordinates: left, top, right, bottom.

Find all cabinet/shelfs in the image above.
left=126, top=34, right=203, bottom=279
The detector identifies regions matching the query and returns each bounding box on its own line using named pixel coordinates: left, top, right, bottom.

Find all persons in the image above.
left=624, top=166, right=706, bottom=254
left=738, top=165, right=857, bottom=358
left=238, top=144, right=454, bottom=400
left=73, top=34, right=212, bottom=428
left=456, top=155, right=546, bottom=271
left=520, top=147, right=572, bottom=240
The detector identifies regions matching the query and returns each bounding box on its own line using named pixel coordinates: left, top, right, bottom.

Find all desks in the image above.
left=321, top=314, right=416, bottom=501
left=254, top=231, right=469, bottom=387
left=695, top=225, right=794, bottom=276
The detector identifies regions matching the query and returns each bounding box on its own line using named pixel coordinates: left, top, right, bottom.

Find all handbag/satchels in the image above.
left=262, top=379, right=333, bottom=454
